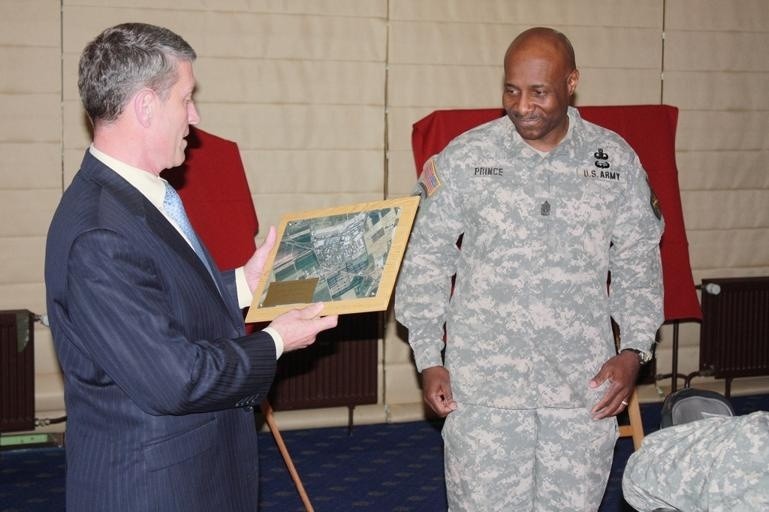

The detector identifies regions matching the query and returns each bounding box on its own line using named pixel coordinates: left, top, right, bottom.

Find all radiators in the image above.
left=0, top=310, right=36, bottom=433
left=699, top=275, right=769, bottom=378
left=242, top=305, right=378, bottom=411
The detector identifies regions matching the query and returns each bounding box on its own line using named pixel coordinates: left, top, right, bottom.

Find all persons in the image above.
left=42, top=22, right=340, bottom=510
left=391, top=25, right=666, bottom=512
left=623, top=406, right=769, bottom=512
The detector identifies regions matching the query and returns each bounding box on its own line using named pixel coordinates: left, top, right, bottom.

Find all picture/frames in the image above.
left=244, top=195, right=423, bottom=324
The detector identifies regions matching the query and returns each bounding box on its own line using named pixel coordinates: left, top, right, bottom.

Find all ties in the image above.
left=161, top=180, right=221, bottom=296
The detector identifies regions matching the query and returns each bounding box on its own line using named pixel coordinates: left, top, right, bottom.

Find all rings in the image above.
left=622, top=401, right=630, bottom=407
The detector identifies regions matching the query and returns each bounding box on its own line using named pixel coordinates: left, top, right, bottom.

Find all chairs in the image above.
left=659, top=388, right=735, bottom=431
